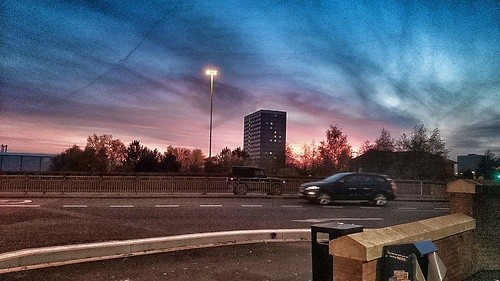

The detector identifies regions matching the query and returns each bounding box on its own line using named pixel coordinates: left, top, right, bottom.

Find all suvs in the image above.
left=297, top=172, right=398, bottom=207
left=225, top=166, right=287, bottom=197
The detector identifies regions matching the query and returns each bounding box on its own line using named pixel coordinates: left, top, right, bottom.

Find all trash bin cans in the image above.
left=383, top=240, right=439, bottom=281
left=310, top=223, right=364, bottom=281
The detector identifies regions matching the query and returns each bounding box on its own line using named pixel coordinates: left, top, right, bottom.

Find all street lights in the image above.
left=205, top=69, right=218, bottom=158
